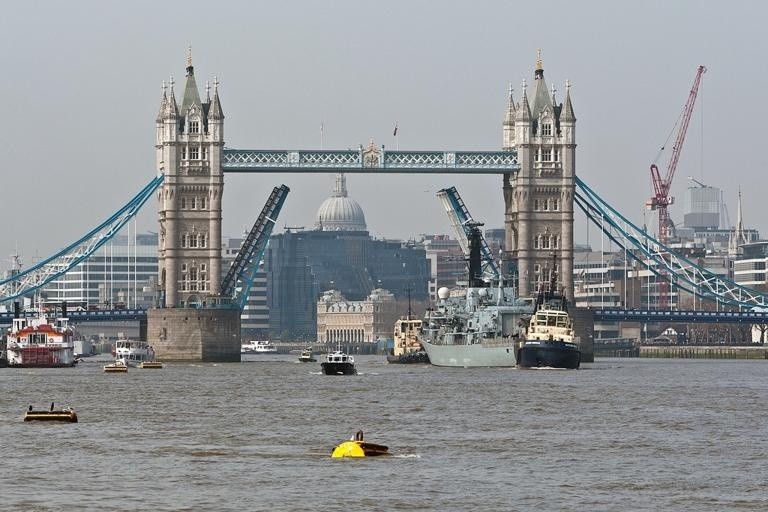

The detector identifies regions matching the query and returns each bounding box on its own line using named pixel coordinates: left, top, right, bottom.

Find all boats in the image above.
left=298, top=346, right=317, bottom=362
left=104, top=339, right=162, bottom=373
left=240, top=340, right=278, bottom=354
left=386, top=220, right=581, bottom=370
left=330, top=429, right=392, bottom=458
left=320, top=350, right=358, bottom=375
left=7, top=314, right=74, bottom=367
left=23, top=401, right=76, bottom=422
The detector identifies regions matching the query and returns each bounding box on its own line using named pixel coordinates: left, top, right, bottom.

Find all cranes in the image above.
left=650, top=65, right=707, bottom=312
